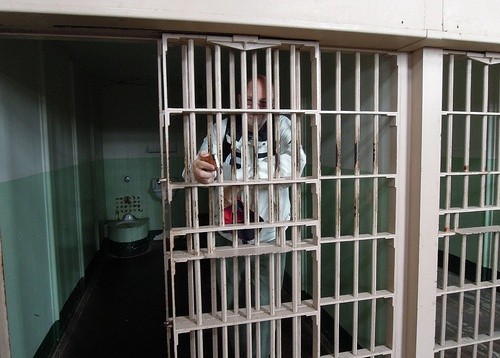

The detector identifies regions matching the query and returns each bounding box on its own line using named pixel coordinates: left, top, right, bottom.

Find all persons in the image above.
left=181, top=74, right=308, bottom=358
